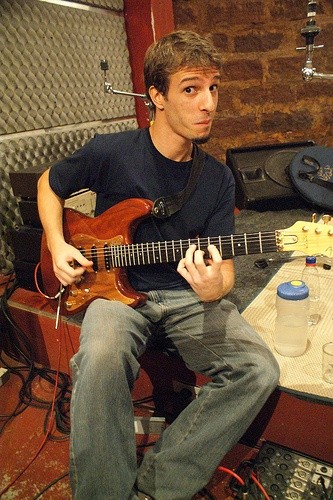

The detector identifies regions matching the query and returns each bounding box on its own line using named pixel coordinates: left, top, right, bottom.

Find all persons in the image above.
left=37, top=31, right=280, bottom=500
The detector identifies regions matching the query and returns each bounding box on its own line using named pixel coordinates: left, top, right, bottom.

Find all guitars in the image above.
left=41, top=198, right=333, bottom=316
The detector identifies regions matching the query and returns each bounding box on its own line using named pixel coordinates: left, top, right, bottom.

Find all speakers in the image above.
left=223, top=141, right=316, bottom=212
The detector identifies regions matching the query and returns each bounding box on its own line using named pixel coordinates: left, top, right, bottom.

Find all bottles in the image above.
left=273, top=280, right=309, bottom=356
left=301, top=256, right=321, bottom=325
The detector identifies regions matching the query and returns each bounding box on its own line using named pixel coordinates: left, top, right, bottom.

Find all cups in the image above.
left=322, top=342, right=333, bottom=383
